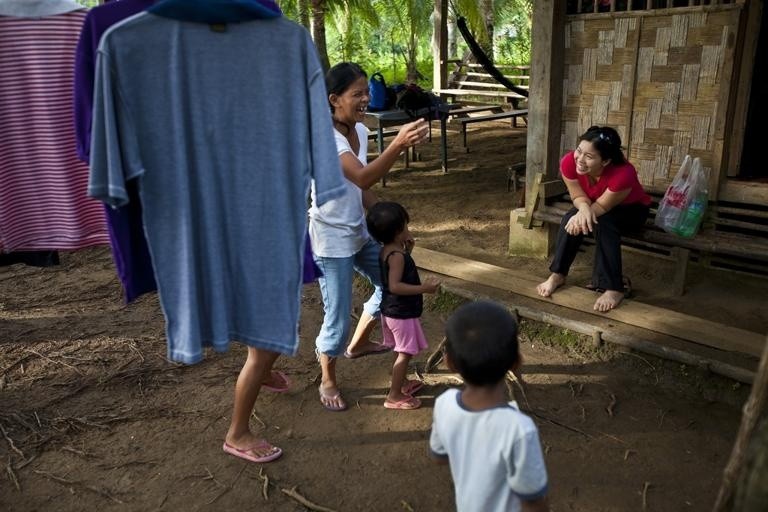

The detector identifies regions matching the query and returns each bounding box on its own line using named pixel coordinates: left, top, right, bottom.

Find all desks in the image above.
left=360, top=102, right=451, bottom=187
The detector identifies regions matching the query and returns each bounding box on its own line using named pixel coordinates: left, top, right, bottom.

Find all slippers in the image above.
left=383, top=392, right=421, bottom=411
left=223, top=437, right=283, bottom=464
left=318, top=384, right=346, bottom=411
left=261, top=369, right=290, bottom=393
left=401, top=379, right=425, bottom=396
left=344, top=341, right=392, bottom=360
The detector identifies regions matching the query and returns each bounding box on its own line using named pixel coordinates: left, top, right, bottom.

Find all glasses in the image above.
left=586, top=126, right=614, bottom=148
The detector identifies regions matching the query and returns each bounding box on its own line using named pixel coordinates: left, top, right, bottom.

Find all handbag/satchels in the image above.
left=367, top=73, right=388, bottom=114
left=395, top=83, right=436, bottom=112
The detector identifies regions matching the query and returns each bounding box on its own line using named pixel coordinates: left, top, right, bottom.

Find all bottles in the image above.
left=676, top=188, right=709, bottom=240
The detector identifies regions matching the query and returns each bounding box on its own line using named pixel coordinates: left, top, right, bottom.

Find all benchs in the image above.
left=439, top=100, right=529, bottom=153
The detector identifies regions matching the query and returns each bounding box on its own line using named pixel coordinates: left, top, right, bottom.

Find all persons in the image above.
left=365, top=202, right=441, bottom=409
left=223, top=346, right=291, bottom=463
left=537, top=126, right=652, bottom=312
left=308, top=61, right=430, bottom=411
left=427, top=300, right=550, bottom=512
